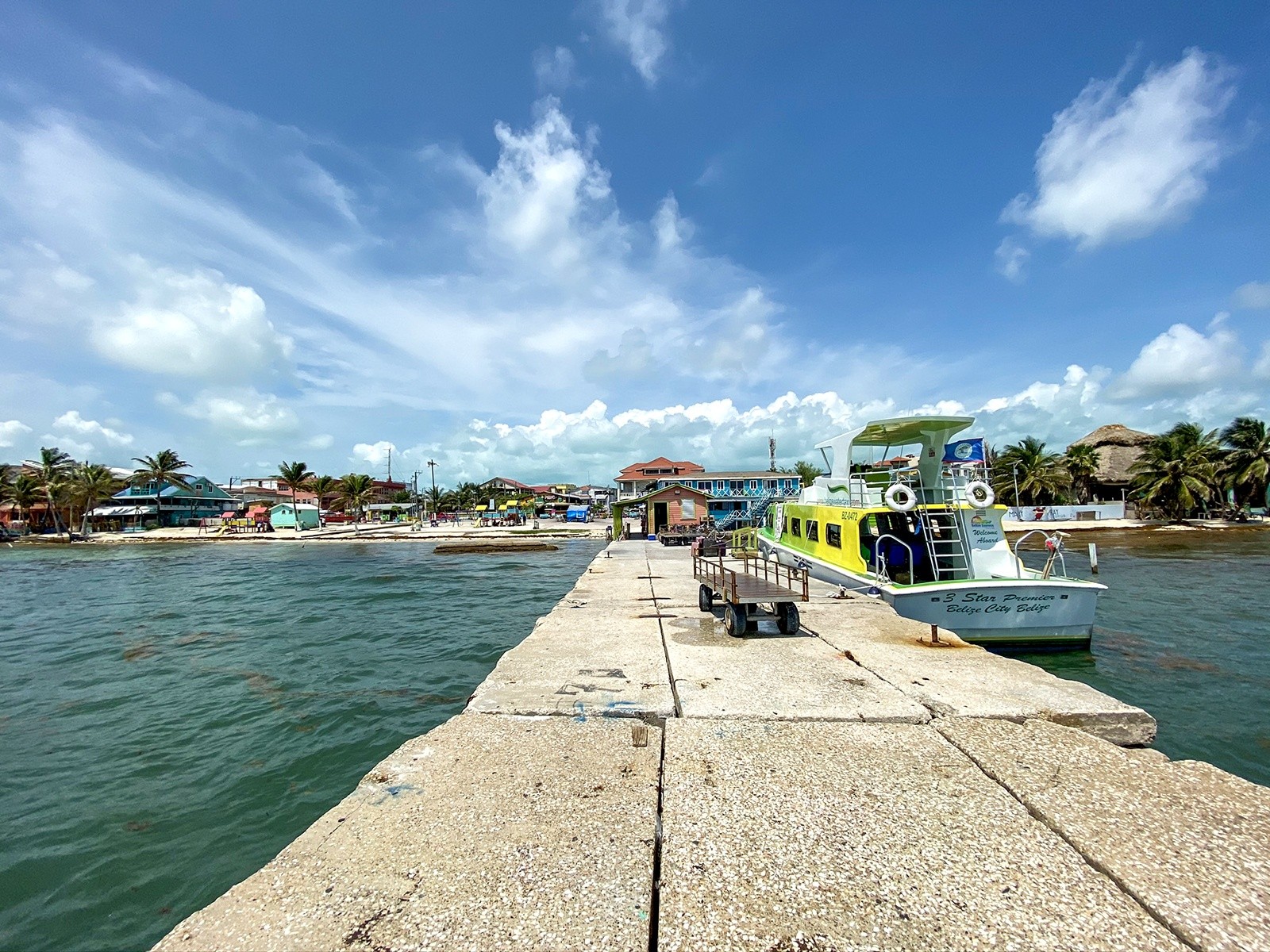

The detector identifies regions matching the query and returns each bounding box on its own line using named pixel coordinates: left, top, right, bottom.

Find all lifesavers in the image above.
left=964, top=480, right=995, bottom=507
left=885, top=484, right=916, bottom=510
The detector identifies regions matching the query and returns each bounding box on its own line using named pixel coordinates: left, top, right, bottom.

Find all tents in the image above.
left=566, top=505, right=591, bottom=522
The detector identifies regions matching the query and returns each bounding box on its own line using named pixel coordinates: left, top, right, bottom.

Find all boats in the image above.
left=750, top=416, right=1107, bottom=647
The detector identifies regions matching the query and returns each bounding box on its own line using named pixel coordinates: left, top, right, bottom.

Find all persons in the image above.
left=322, top=516, right=326, bottom=527
left=506, top=513, right=514, bottom=526
left=523, top=514, right=526, bottom=525
left=256, top=521, right=268, bottom=533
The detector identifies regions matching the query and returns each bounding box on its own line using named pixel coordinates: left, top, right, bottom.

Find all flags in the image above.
left=942, top=437, right=985, bottom=466
